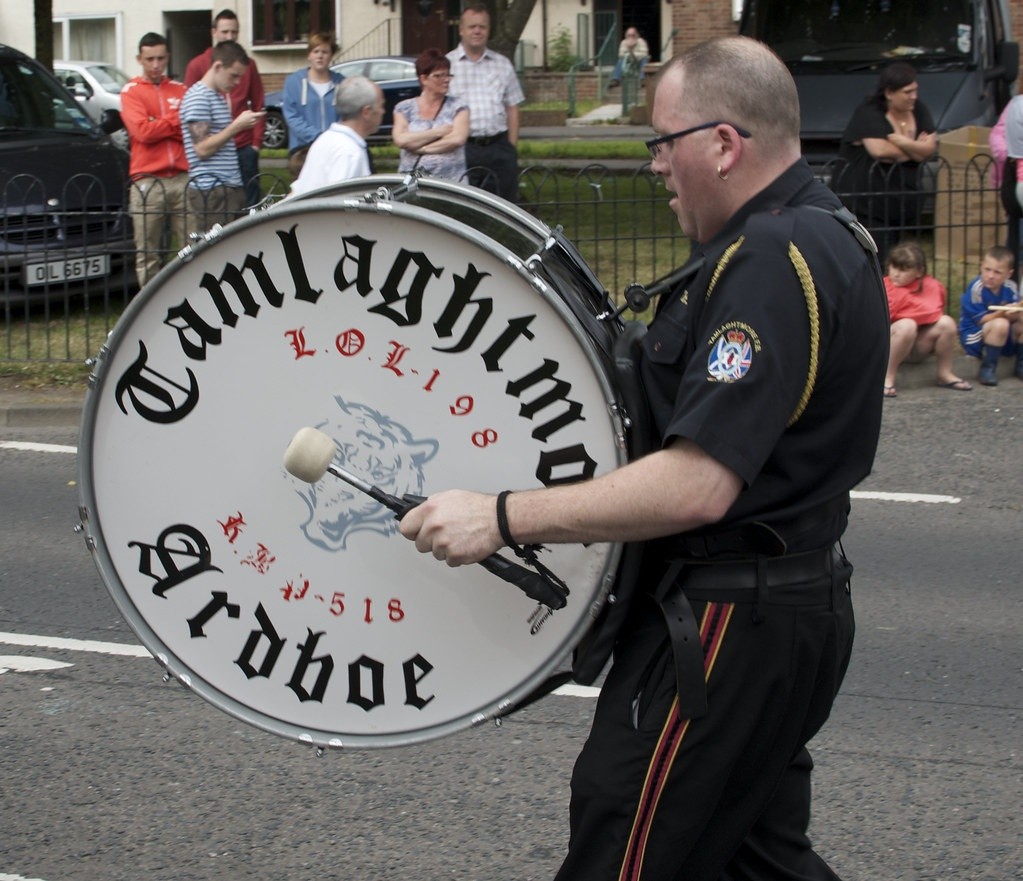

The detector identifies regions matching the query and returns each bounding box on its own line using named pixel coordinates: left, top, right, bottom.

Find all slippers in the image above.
left=938, top=375, right=974, bottom=390
left=884, top=386, right=896, bottom=396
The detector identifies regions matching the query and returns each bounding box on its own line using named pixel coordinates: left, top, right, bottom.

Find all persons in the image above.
left=400, top=36, right=892, bottom=881
left=988, top=55, right=1023, bottom=301
left=391, top=49, right=472, bottom=187
left=289, top=74, right=386, bottom=202
left=185, top=9, right=267, bottom=214
left=876, top=242, right=971, bottom=397
left=281, top=34, right=344, bottom=182
left=829, top=63, right=939, bottom=276
left=119, top=31, right=189, bottom=290
left=179, top=39, right=267, bottom=248
left=957, top=244, right=1023, bottom=386
left=441, top=7, right=525, bottom=205
left=606, top=27, right=651, bottom=89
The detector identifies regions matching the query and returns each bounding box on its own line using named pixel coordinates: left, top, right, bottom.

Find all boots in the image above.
left=978, top=343, right=1002, bottom=384
left=1014, top=343, right=1023, bottom=378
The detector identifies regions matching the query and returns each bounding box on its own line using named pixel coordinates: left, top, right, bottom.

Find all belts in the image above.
left=631, top=539, right=845, bottom=720
left=467, top=130, right=508, bottom=146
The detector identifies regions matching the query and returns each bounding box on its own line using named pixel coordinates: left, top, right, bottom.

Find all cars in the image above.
left=737, top=1, right=1020, bottom=236
left=33, top=57, right=131, bottom=146
left=257, top=56, right=454, bottom=153
left=0, top=42, right=141, bottom=317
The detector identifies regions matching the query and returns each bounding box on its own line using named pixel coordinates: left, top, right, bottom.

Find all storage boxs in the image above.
left=933, top=124, right=1008, bottom=265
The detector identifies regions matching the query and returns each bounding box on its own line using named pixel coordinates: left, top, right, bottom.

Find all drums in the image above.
left=78, top=172, right=641, bottom=757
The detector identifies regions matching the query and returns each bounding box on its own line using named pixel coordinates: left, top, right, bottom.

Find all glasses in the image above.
left=645, top=119, right=752, bottom=161
left=426, top=73, right=453, bottom=79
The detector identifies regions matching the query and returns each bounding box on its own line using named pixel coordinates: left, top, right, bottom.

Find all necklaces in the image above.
left=901, top=122, right=907, bottom=126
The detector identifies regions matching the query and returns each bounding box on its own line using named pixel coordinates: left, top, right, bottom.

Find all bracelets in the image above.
left=497, top=491, right=546, bottom=561
